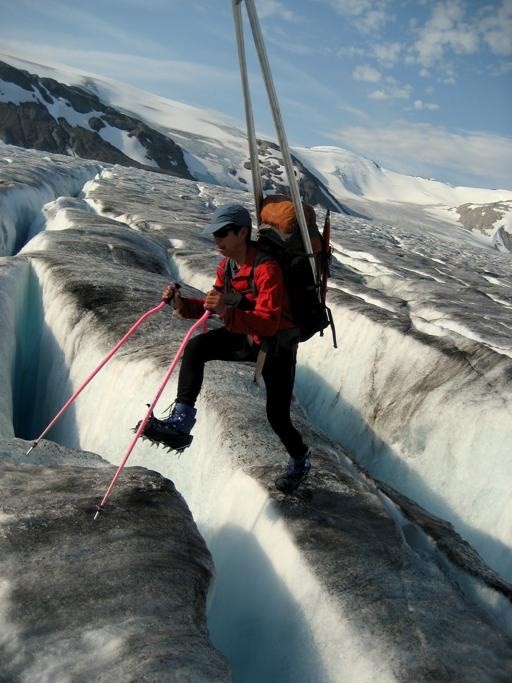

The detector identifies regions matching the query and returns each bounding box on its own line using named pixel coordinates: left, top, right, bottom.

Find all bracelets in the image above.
left=219, top=304, right=228, bottom=317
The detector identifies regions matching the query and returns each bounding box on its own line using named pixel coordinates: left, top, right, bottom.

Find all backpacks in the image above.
left=247, top=194, right=337, bottom=348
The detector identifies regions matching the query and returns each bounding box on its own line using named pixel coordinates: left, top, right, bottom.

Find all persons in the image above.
left=136, top=201, right=312, bottom=495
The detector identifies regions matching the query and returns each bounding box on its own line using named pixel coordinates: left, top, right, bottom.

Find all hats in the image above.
left=200, top=204, right=251, bottom=235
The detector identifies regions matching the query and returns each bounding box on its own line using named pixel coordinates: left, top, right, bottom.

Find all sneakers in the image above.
left=275, top=448, right=311, bottom=493
left=136, top=403, right=196, bottom=451
